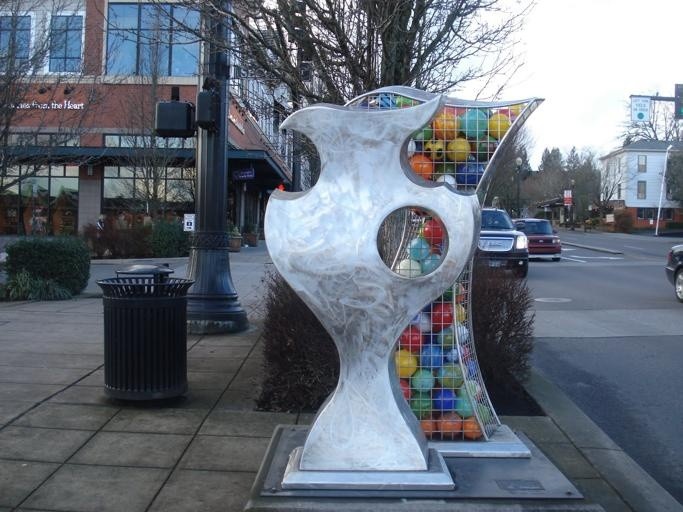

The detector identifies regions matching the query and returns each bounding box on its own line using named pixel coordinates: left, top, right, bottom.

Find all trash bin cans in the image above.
left=94, top=264, right=196, bottom=401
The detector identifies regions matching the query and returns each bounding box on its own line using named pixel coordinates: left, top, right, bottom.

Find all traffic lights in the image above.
left=155, top=101, right=195, bottom=136
left=197, top=92, right=220, bottom=129
left=675, top=84, right=683, bottom=119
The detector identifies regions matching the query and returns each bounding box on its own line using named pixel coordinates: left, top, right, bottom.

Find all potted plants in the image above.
left=229, top=224, right=242, bottom=252
left=244, top=222, right=260, bottom=248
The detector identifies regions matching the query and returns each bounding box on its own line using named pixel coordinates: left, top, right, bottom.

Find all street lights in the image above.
left=571, top=180, right=575, bottom=224
left=516, top=157, right=522, bottom=217
left=655, top=145, right=674, bottom=236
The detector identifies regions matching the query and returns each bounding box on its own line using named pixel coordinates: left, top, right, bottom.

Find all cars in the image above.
left=664, top=244, right=683, bottom=303
left=477, top=208, right=561, bottom=278
